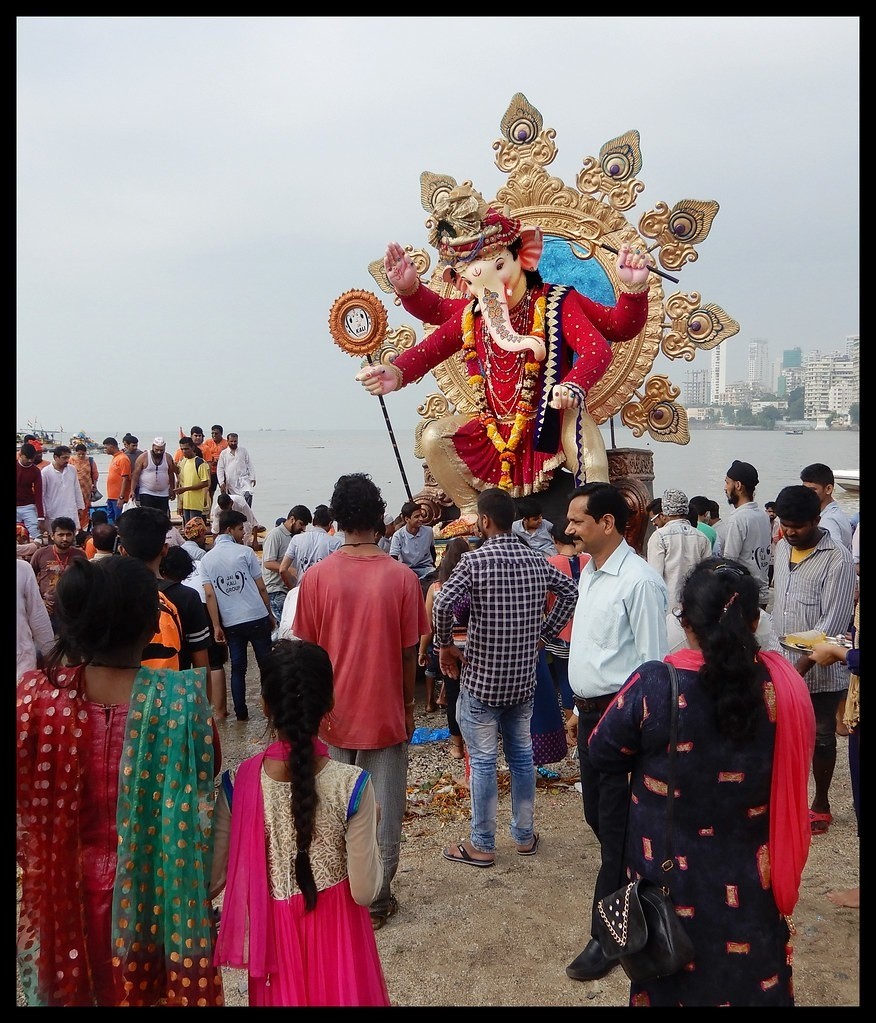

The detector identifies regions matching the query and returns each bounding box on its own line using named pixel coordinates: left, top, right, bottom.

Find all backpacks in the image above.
left=139, top=580, right=187, bottom=671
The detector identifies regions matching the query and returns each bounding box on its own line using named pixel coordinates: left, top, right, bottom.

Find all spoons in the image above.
left=795, top=643, right=811, bottom=647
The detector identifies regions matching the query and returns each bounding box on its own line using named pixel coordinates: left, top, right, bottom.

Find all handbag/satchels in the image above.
left=122, top=499, right=138, bottom=513
left=89, top=485, right=103, bottom=502
left=596, top=877, right=695, bottom=982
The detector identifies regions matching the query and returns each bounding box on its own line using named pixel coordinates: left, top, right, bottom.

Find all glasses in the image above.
left=650, top=514, right=661, bottom=522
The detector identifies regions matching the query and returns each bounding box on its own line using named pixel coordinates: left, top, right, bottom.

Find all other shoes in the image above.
left=211, top=904, right=224, bottom=927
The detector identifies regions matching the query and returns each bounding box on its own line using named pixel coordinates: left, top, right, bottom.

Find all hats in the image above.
left=727, top=460, right=759, bottom=488
left=184, top=517, right=207, bottom=539
left=153, top=437, right=166, bottom=447
left=661, top=489, right=689, bottom=516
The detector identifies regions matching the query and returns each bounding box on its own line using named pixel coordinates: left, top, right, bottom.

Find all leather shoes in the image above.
left=567, top=939, right=620, bottom=981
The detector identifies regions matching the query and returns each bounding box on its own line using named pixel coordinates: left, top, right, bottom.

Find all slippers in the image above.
left=443, top=845, right=494, bottom=866
left=517, top=831, right=539, bottom=855
left=807, top=807, right=832, bottom=836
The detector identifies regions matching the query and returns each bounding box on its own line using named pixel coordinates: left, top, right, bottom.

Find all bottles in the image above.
left=787, top=630, right=825, bottom=645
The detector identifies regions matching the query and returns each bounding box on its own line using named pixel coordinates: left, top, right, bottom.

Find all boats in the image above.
left=785, top=429, right=803, bottom=435
left=832, top=469, right=860, bottom=493
left=18, top=416, right=66, bottom=454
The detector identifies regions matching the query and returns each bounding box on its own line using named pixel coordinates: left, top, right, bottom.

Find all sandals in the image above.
left=367, top=893, right=397, bottom=929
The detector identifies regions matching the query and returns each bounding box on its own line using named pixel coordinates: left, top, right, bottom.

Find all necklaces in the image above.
left=340, top=543, right=379, bottom=547
left=52, top=545, right=72, bottom=573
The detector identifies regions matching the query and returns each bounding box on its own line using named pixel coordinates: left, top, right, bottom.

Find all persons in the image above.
left=116, top=507, right=231, bottom=720
left=290, top=473, right=431, bottom=930
left=17, top=524, right=40, bottom=561
left=16, top=425, right=267, bottom=550
left=588, top=543, right=815, bottom=1007
left=262, top=505, right=431, bottom=642
left=200, top=510, right=276, bottom=722
left=210, top=637, right=391, bottom=1006
left=353, top=180, right=650, bottom=538
left=417, top=482, right=860, bottom=867
left=29, top=517, right=88, bottom=616
left=724, top=459, right=771, bottom=612
left=17, top=556, right=222, bottom=1006
left=800, top=463, right=853, bottom=554
left=563, top=483, right=672, bottom=982
